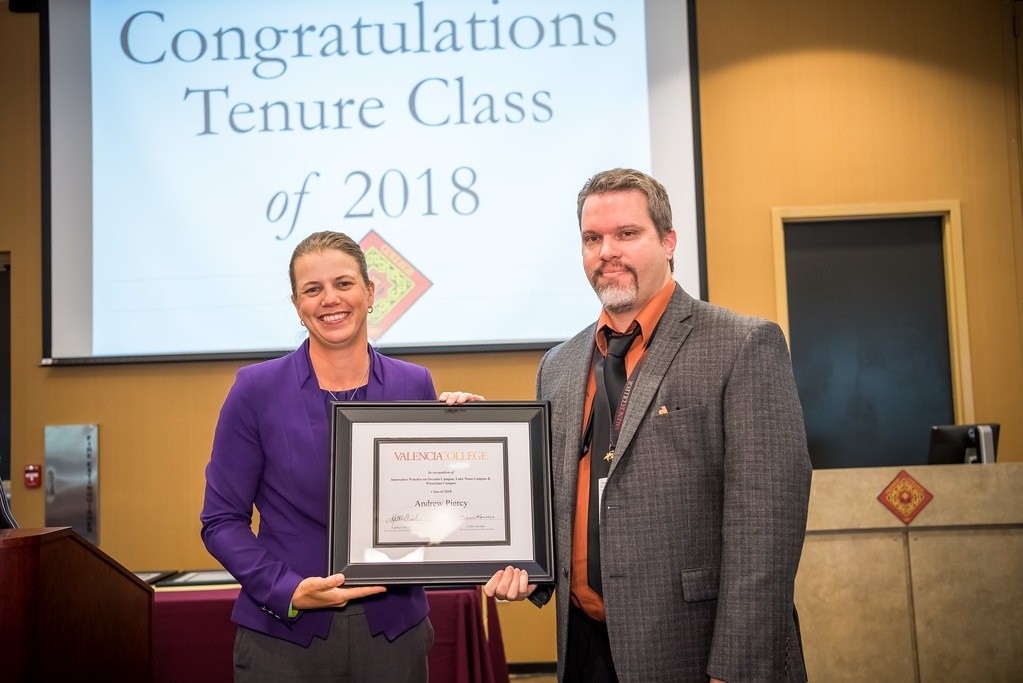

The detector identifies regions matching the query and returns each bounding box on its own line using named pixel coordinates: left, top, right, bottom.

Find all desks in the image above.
left=154, top=582, right=512, bottom=683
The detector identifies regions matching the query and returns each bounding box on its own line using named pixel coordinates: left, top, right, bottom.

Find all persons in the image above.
left=200, top=230, right=485, bottom=683
left=481, top=168, right=809, bottom=683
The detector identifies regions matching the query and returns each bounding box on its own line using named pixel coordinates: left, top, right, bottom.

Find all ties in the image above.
left=579, top=323, right=644, bottom=598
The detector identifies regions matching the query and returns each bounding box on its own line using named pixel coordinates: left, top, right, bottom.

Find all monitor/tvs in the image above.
left=928, top=423, right=999, bottom=464
